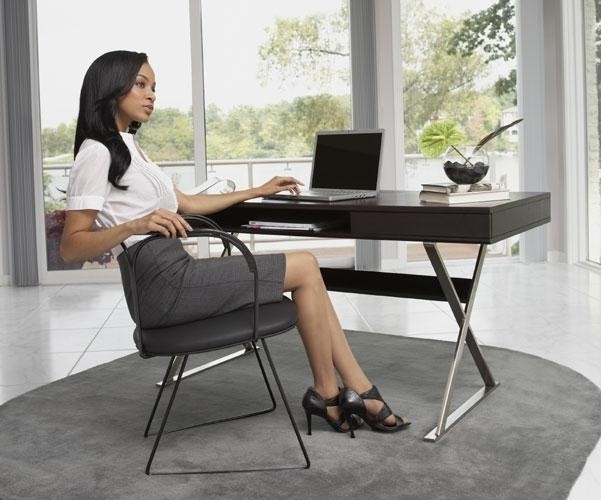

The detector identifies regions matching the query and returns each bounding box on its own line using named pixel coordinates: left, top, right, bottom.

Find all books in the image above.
left=419, top=189, right=511, bottom=205
left=247, top=220, right=325, bottom=231
left=420, top=182, right=459, bottom=194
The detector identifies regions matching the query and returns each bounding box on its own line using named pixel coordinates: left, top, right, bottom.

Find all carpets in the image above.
left=0, top=324, right=601, bottom=496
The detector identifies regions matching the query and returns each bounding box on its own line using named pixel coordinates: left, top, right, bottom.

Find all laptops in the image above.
left=265, top=128, right=388, bottom=203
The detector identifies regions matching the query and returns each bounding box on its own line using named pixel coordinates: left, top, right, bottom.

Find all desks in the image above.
left=196, top=182, right=552, bottom=446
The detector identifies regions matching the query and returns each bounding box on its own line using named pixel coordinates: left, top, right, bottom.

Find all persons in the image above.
left=57, top=49, right=412, bottom=440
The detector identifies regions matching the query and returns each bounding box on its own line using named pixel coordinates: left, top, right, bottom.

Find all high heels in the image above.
left=302, top=386, right=365, bottom=435
left=340, top=386, right=410, bottom=438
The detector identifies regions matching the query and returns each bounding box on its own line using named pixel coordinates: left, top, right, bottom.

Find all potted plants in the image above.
left=413, top=114, right=528, bottom=183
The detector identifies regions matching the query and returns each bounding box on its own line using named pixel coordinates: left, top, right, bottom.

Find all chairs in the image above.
left=115, top=211, right=312, bottom=472
